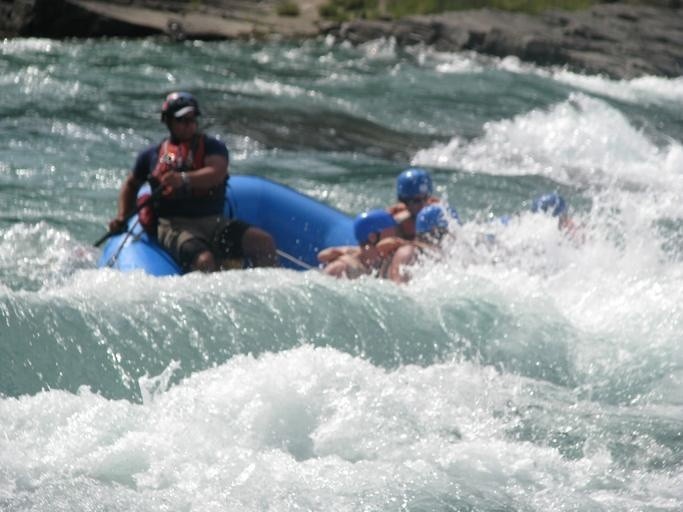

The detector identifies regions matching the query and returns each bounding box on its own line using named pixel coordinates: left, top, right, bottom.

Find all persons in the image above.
left=108, top=90, right=278, bottom=273
left=316, top=168, right=582, bottom=292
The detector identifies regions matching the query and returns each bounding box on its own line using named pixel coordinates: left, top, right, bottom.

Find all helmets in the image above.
left=531, top=193, right=566, bottom=219
left=161, top=91, right=201, bottom=119
left=353, top=209, right=402, bottom=243
left=396, top=168, right=433, bottom=201
left=414, top=205, right=461, bottom=234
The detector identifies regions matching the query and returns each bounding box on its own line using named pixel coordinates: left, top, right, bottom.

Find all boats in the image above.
left=100, top=172, right=357, bottom=274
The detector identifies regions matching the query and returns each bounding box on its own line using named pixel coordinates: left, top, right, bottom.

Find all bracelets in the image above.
left=181, top=172, right=188, bottom=183
